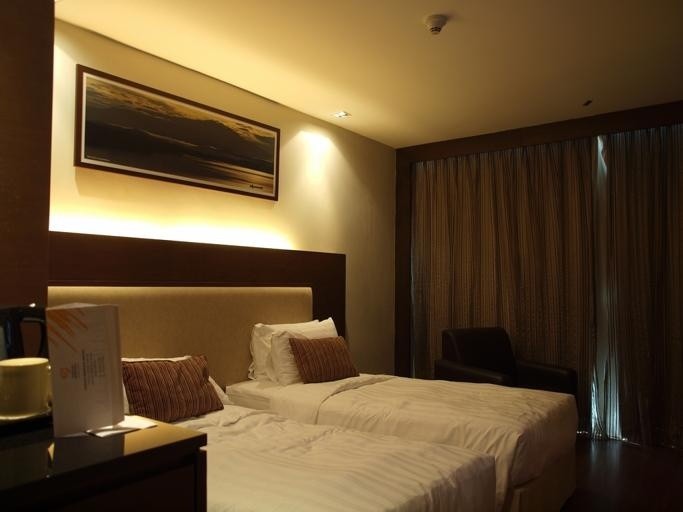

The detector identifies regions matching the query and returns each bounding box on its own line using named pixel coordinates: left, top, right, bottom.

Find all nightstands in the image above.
left=0, top=415, right=209, bottom=512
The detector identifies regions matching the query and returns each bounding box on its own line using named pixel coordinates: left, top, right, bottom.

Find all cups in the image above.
left=1, top=357, right=52, bottom=420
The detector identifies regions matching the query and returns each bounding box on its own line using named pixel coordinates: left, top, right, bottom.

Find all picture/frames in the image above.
left=74, top=62, right=280, bottom=200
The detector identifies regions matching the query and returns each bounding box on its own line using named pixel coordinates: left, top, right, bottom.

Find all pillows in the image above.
left=120, top=352, right=234, bottom=423
left=247, top=316, right=360, bottom=384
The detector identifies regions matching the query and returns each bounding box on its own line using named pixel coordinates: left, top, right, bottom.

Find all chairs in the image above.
left=434, top=327, right=577, bottom=393
